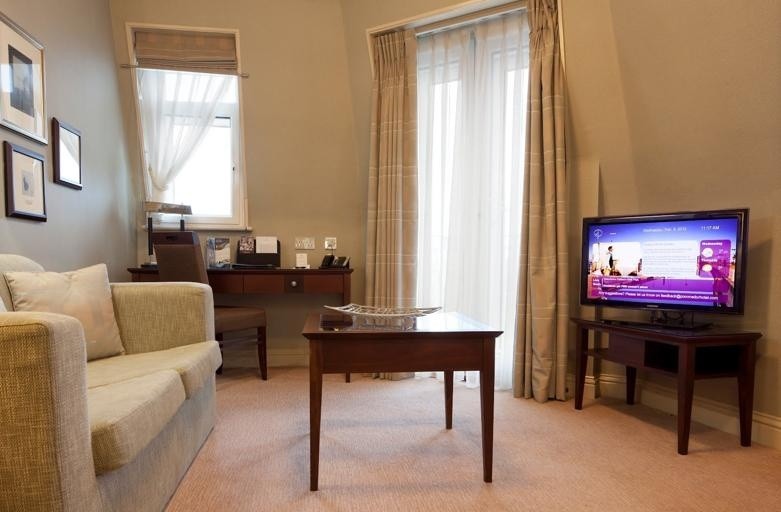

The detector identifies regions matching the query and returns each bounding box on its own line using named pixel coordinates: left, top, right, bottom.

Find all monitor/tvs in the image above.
left=579, top=207, right=750, bottom=329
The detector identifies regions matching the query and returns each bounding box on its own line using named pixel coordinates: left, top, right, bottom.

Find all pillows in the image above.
left=3, top=262, right=124, bottom=361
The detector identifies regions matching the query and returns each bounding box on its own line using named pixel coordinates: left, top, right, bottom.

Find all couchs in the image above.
left=0, top=253, right=223, bottom=512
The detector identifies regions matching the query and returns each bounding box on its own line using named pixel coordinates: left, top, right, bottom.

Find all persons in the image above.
left=605, top=245, right=614, bottom=270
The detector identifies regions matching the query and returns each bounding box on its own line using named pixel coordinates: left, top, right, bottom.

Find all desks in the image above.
left=574, top=316, right=763, bottom=457
left=302, top=310, right=503, bottom=492
left=125, top=263, right=354, bottom=382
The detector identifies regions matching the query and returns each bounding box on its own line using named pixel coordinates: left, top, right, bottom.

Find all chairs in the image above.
left=152, top=230, right=268, bottom=380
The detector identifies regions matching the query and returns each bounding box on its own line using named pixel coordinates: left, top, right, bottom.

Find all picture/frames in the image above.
left=51, top=116, right=83, bottom=189
left=4, top=140, right=48, bottom=222
left=0, top=11, right=48, bottom=146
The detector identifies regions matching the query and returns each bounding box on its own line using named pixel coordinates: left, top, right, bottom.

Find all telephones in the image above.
left=317, top=254, right=351, bottom=270
